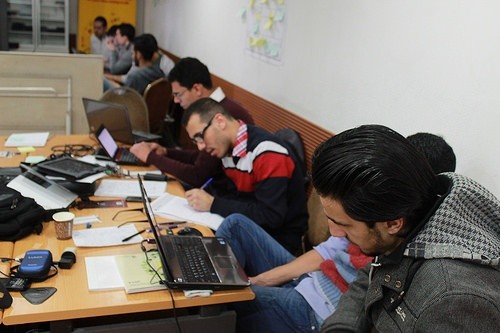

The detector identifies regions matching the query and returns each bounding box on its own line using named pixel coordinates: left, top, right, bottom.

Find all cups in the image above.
left=53, top=212, right=75, bottom=240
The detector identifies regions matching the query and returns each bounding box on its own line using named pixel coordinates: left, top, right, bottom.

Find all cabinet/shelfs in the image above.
left=6, top=0, right=70, bottom=54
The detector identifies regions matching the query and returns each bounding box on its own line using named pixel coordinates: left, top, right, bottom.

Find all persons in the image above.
left=129, top=57, right=254, bottom=187
left=73, top=15, right=174, bottom=97
left=183, top=96, right=309, bottom=255
left=310, top=125, right=500, bottom=333
left=214, top=213, right=376, bottom=333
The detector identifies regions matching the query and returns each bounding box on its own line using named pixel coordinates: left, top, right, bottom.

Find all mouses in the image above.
left=177, top=227, right=203, bottom=236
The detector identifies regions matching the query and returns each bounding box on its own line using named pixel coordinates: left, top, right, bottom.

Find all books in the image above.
left=116, top=250, right=169, bottom=293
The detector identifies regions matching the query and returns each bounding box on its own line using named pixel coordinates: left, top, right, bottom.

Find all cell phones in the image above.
left=0, top=278, right=31, bottom=291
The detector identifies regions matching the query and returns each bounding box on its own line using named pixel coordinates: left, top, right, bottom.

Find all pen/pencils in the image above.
left=199, top=177, right=213, bottom=190
left=159, top=221, right=187, bottom=225
left=123, top=229, right=146, bottom=241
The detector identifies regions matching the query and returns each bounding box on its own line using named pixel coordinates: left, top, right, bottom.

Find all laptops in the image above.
left=95, top=123, right=149, bottom=164
left=138, top=172, right=253, bottom=289
left=80, top=98, right=164, bottom=144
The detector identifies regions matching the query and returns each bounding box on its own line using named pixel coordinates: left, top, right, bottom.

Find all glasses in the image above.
left=193, top=112, right=225, bottom=144
left=112, top=208, right=149, bottom=228
left=172, top=87, right=189, bottom=97
left=51, top=144, right=89, bottom=152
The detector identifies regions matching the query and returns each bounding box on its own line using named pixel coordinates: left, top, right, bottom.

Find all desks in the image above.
left=0, top=133, right=255, bottom=333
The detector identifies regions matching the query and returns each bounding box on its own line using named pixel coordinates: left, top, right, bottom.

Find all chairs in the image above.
left=143, top=78, right=172, bottom=128
left=100, top=87, right=149, bottom=133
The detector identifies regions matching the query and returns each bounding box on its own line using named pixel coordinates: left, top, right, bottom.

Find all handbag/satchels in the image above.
left=0, top=191, right=47, bottom=243
